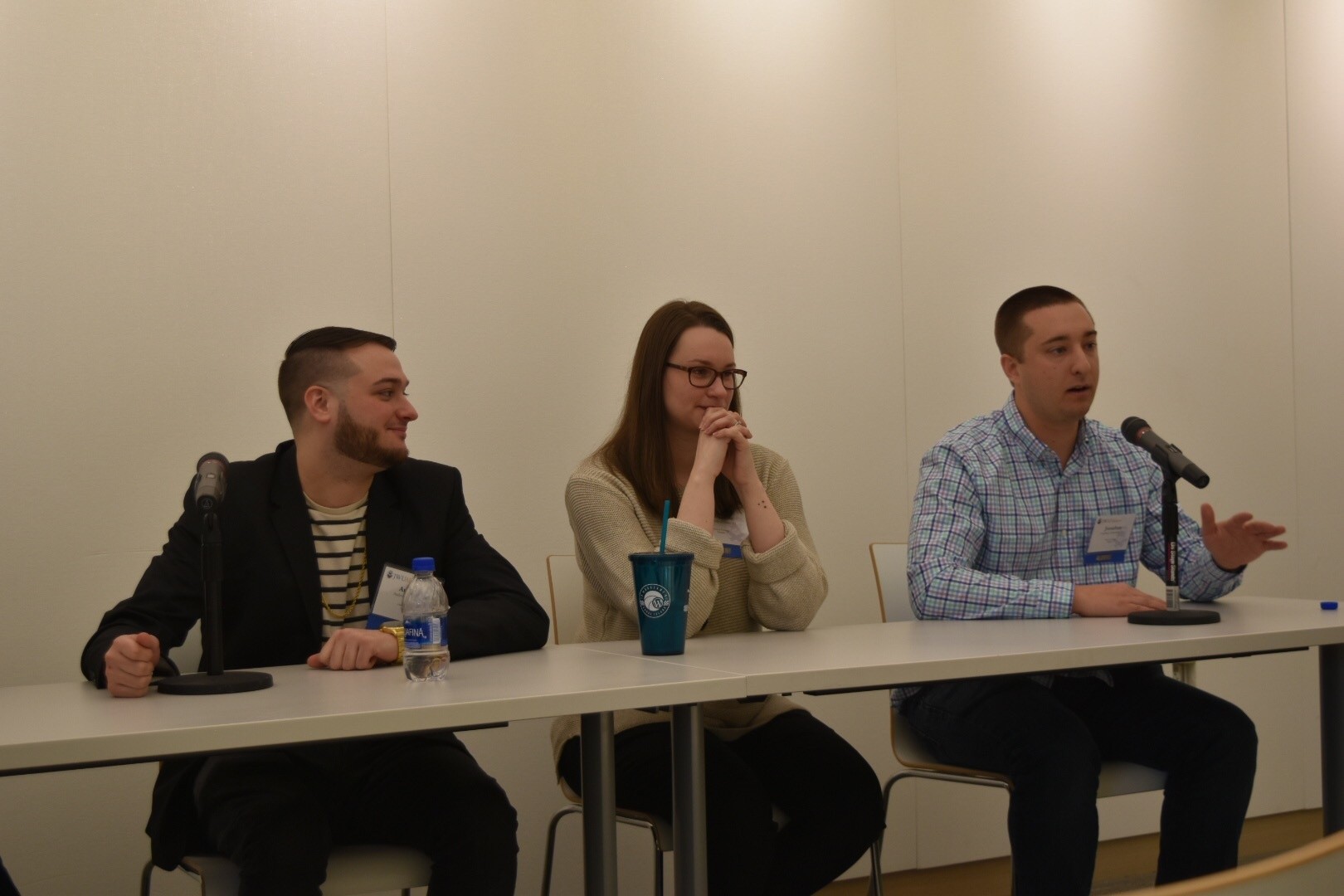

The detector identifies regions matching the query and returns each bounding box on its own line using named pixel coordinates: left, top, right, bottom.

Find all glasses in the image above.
left=667, top=361, right=748, bottom=390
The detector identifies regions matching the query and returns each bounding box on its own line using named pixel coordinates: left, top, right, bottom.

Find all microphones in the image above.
left=1120, top=414, right=1223, bottom=492
left=154, top=452, right=274, bottom=517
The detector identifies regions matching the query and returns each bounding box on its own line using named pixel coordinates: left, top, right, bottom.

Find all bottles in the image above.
left=400, top=557, right=450, bottom=680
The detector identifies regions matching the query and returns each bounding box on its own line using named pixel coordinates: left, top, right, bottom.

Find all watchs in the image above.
left=379, top=621, right=406, bottom=665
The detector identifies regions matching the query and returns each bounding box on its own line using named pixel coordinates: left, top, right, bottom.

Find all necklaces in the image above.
left=322, top=546, right=367, bottom=618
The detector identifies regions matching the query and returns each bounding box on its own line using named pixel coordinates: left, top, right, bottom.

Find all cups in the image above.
left=629, top=551, right=695, bottom=656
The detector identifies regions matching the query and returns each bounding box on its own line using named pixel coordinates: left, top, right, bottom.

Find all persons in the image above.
left=565, top=301, right=885, bottom=895
left=82, top=324, right=550, bottom=896
left=895, top=285, right=1291, bottom=896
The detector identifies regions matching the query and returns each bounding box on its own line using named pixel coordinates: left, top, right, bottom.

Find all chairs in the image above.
left=866, top=543, right=1166, bottom=896
left=539, top=555, right=882, bottom=896
left=138, top=622, right=431, bottom=896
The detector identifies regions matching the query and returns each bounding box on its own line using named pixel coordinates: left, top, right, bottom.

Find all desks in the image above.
left=0, top=645, right=744, bottom=896
left=573, top=595, right=1344, bottom=896
